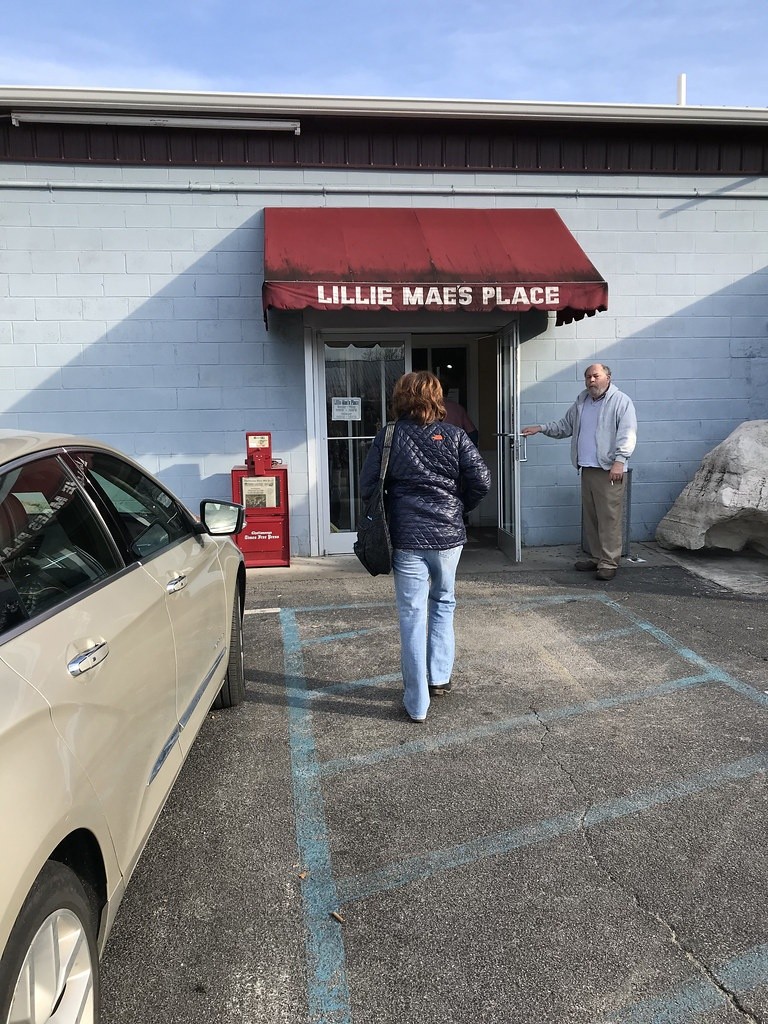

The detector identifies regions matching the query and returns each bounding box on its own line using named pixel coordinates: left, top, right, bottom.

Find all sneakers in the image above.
left=430, top=677, right=452, bottom=696
left=574, top=560, right=598, bottom=571
left=592, top=566, right=617, bottom=580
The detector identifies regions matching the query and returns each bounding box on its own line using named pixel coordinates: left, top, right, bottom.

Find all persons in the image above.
left=521, top=364, right=638, bottom=580
left=354, top=368, right=490, bottom=724
left=439, top=378, right=480, bottom=448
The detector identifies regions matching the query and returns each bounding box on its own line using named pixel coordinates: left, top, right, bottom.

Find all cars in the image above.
left=0, top=423, right=256, bottom=1024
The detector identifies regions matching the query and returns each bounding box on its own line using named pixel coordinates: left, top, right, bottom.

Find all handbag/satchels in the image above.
left=352, top=487, right=394, bottom=577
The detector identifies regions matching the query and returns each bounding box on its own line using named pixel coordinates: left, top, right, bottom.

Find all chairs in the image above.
left=0, top=493, right=106, bottom=619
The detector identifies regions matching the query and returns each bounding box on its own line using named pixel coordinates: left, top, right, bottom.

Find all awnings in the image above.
left=261, top=204, right=608, bottom=332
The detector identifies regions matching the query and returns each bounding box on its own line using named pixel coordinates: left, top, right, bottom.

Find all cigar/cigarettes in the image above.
left=611, top=480, right=614, bottom=485
